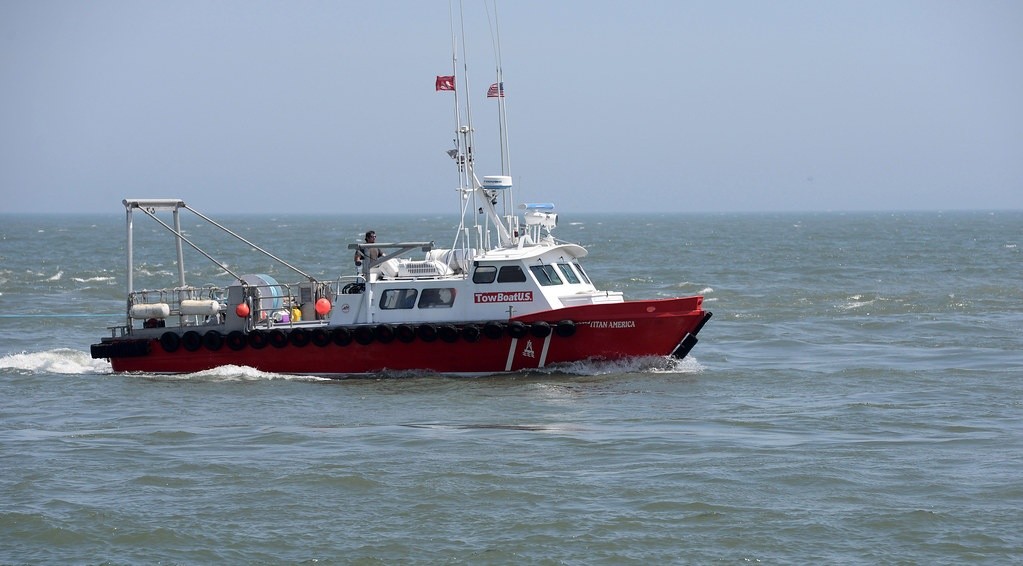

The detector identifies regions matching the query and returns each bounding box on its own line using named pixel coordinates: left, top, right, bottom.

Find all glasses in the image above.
left=370, top=236, right=376, bottom=238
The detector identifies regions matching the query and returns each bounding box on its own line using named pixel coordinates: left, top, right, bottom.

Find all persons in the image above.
left=355, top=231, right=387, bottom=266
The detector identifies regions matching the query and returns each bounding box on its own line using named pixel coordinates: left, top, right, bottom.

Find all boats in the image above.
left=89, top=0, right=714, bottom=378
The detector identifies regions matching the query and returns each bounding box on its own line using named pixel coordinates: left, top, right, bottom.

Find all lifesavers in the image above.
left=530, top=321, right=551, bottom=338
left=439, top=323, right=458, bottom=343
left=268, top=328, right=288, bottom=348
left=556, top=319, right=576, bottom=337
left=247, top=329, right=268, bottom=350
left=460, top=324, right=482, bottom=343
left=507, top=321, right=527, bottom=338
left=484, top=320, right=503, bottom=339
left=418, top=322, right=438, bottom=343
left=202, top=330, right=224, bottom=351
left=396, top=323, right=416, bottom=342
left=226, top=330, right=247, bottom=350
left=353, top=325, right=374, bottom=345
left=159, top=331, right=181, bottom=352
left=311, top=327, right=331, bottom=347
left=288, top=328, right=309, bottom=347
left=332, top=326, right=352, bottom=346
left=180, top=330, right=202, bottom=352
left=375, top=323, right=395, bottom=343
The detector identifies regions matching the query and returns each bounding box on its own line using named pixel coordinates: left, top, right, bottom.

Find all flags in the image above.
left=435, top=75, right=455, bottom=91
left=487, top=82, right=504, bottom=97
